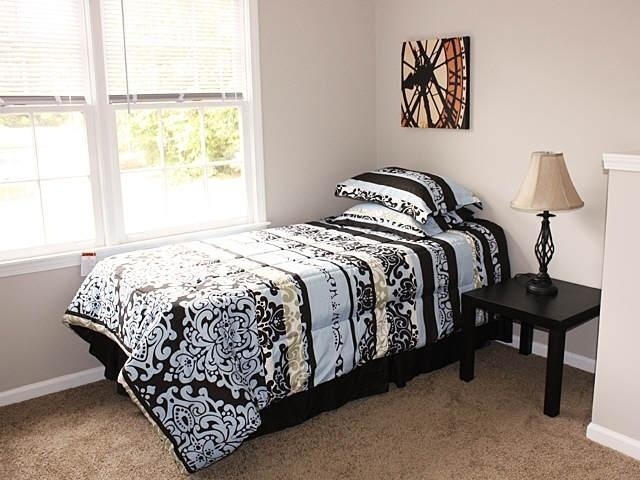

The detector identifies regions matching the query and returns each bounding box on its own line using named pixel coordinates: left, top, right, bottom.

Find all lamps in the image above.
left=510, top=151, right=586, bottom=297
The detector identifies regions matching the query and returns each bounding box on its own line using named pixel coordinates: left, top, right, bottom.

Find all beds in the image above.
left=61, top=215, right=512, bottom=473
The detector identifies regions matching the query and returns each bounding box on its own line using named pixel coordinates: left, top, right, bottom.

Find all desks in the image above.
left=458, top=272, right=601, bottom=417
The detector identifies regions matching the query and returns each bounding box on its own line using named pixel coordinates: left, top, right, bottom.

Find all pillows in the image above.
left=326, top=203, right=475, bottom=242
left=333, top=166, right=484, bottom=226
